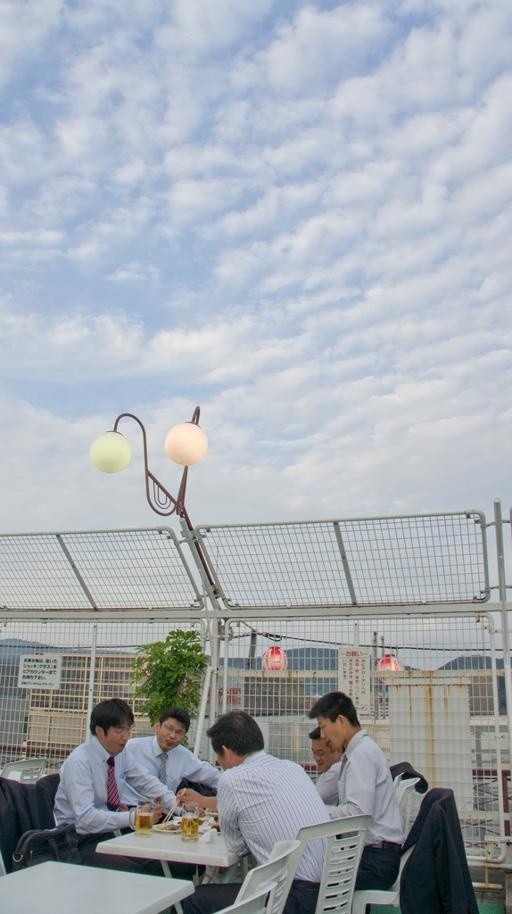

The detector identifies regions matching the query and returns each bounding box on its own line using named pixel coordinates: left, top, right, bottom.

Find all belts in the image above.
left=364, top=841, right=401, bottom=853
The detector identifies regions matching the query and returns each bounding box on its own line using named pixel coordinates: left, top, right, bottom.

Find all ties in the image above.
left=336, top=754, right=347, bottom=807
left=107, top=755, right=121, bottom=813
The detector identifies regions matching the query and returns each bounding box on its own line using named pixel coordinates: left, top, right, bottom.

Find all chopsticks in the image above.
left=162, top=788, right=187, bottom=823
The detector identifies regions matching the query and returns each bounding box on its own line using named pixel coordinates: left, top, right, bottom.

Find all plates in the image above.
left=154, top=811, right=219, bottom=835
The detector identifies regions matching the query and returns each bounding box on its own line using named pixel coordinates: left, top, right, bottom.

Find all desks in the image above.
left=1, top=824, right=242, bottom=914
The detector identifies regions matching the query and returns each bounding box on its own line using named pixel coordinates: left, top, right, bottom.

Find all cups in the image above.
left=181, top=802, right=200, bottom=840
left=129, top=799, right=154, bottom=834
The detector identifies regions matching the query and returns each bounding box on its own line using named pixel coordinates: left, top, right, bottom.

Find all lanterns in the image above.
left=261, top=644, right=289, bottom=673
left=374, top=652, right=402, bottom=684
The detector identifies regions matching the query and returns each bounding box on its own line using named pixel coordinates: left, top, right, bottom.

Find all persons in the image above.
left=307, top=726, right=342, bottom=770
left=165, top=710, right=344, bottom=914
left=55, top=698, right=188, bottom=877
left=309, top=691, right=405, bottom=891
left=122, top=706, right=221, bottom=833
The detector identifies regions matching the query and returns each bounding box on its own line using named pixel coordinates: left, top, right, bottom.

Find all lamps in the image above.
left=88, top=407, right=221, bottom=602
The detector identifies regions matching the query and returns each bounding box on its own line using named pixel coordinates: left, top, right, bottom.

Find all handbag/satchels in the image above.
left=11, top=822, right=82, bottom=875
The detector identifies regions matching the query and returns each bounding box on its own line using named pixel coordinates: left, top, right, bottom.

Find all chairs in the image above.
left=0, top=757, right=46, bottom=785
left=214, top=761, right=452, bottom=914
